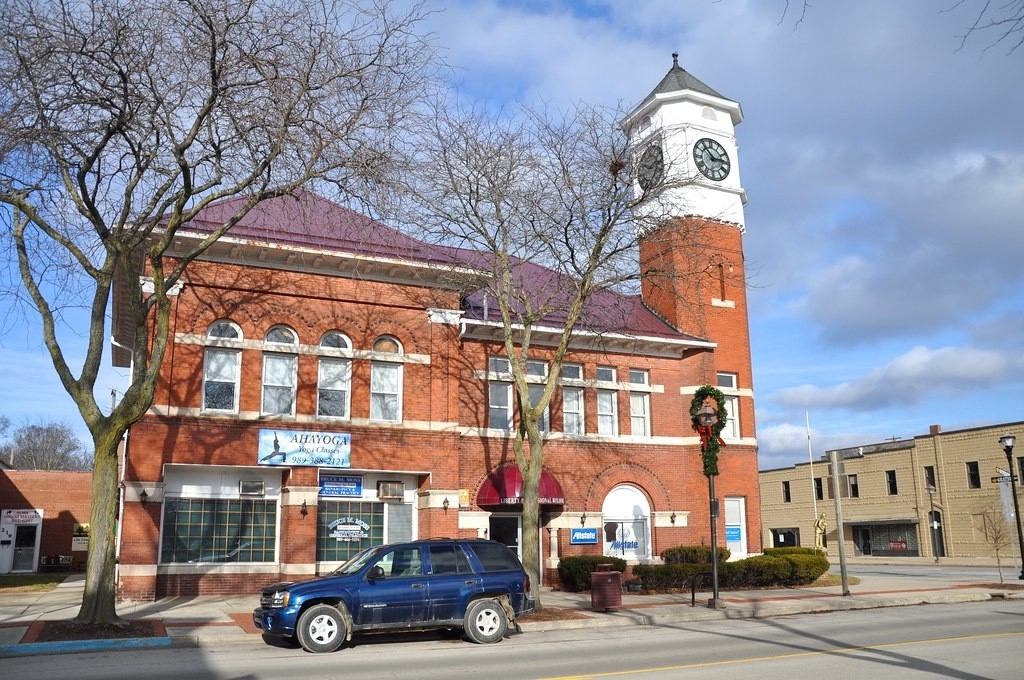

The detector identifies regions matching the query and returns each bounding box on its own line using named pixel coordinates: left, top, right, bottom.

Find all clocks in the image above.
left=637, top=145, right=663, bottom=191
left=693, top=138, right=731, bottom=181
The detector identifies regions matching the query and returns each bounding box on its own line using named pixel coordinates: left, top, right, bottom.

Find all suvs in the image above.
left=251, top=536, right=536, bottom=654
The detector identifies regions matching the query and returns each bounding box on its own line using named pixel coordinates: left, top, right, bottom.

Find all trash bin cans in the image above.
left=590, top=563, right=622, bottom=611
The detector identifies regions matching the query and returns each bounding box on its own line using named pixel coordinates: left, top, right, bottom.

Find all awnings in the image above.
left=477, top=463, right=564, bottom=505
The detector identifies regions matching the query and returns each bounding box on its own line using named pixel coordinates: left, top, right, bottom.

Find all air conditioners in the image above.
left=240, top=479, right=265, bottom=495
left=379, top=482, right=405, bottom=498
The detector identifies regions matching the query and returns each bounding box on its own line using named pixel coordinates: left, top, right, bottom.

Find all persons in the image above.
left=813, top=513, right=827, bottom=549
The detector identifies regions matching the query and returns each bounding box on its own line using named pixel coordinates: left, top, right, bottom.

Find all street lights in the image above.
left=998, top=433, right=1024, bottom=580
left=925, top=483, right=939, bottom=559
left=696, top=398, right=721, bottom=598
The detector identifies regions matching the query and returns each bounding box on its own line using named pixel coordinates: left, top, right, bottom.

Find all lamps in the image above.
left=443, top=498, right=450, bottom=515
left=580, top=513, right=587, bottom=527
left=671, top=512, right=676, bottom=523
left=139, top=486, right=148, bottom=508
left=301, top=499, right=308, bottom=519
